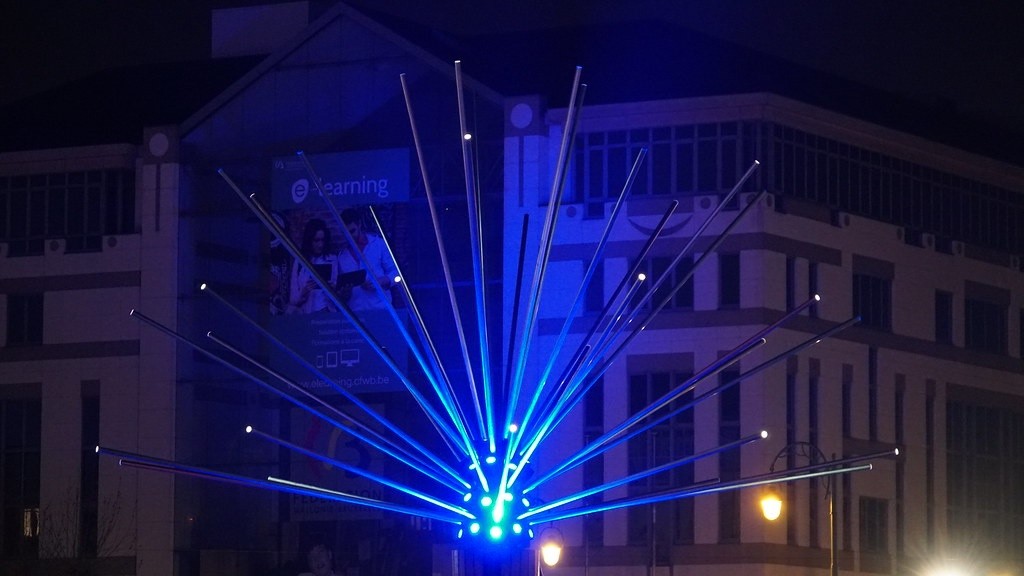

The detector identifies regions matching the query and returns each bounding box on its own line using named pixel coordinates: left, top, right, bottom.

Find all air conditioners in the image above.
left=759, top=194, right=774, bottom=209
left=103, top=235, right=124, bottom=251
left=44, top=239, right=67, bottom=256
left=606, top=200, right=627, bottom=218
left=740, top=193, right=761, bottom=209
left=1007, top=255, right=1021, bottom=271
left=918, top=234, right=937, bottom=250
left=949, top=241, right=969, bottom=256
left=696, top=194, right=720, bottom=213
left=838, top=212, right=856, bottom=229
left=558, top=203, right=588, bottom=223
left=894, top=226, right=905, bottom=243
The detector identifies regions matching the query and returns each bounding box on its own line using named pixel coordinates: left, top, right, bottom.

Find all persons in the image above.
left=289, top=218, right=339, bottom=315
left=333, top=207, right=398, bottom=309
left=292, top=533, right=339, bottom=576
